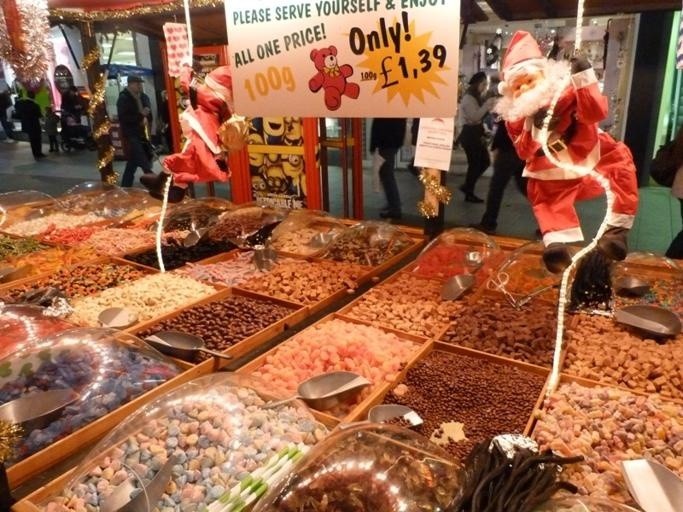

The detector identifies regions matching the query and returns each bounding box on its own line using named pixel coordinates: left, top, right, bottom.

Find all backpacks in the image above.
left=650, top=129, right=682, bottom=188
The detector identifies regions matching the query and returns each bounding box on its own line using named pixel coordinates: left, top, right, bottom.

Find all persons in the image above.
left=66, top=117, right=79, bottom=126
left=45, top=107, right=60, bottom=152
left=161, top=89, right=171, bottom=155
left=140, top=66, right=232, bottom=204
left=61, top=86, right=80, bottom=129
left=455, top=72, right=499, bottom=204
left=494, top=30, right=638, bottom=273
left=0, top=100, right=16, bottom=143
left=370, top=118, right=407, bottom=224
left=14, top=90, right=47, bottom=160
left=116, top=75, right=156, bottom=187
left=469, top=120, right=542, bottom=239
left=665, top=124, right=683, bottom=260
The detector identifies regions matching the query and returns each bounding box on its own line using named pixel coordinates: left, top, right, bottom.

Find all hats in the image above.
left=205, top=66, right=237, bottom=111
left=502, top=31, right=542, bottom=68
left=128, top=73, right=146, bottom=83
